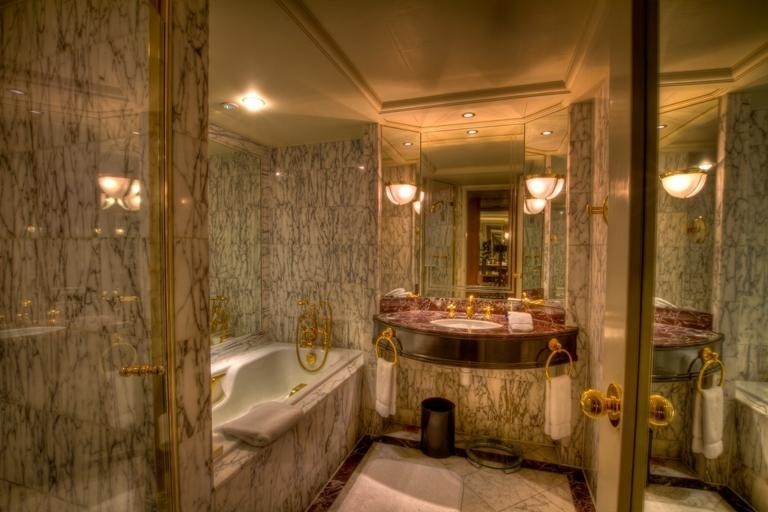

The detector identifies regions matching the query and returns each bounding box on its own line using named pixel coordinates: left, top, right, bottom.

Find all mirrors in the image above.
left=637, top=2, right=768, bottom=512
left=377, top=105, right=577, bottom=313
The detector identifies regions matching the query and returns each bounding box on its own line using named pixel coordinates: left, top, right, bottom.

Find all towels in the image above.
left=212, top=399, right=304, bottom=448
left=373, top=357, right=399, bottom=419
left=544, top=373, right=574, bottom=441
left=691, top=386, right=724, bottom=459
left=102, top=368, right=144, bottom=430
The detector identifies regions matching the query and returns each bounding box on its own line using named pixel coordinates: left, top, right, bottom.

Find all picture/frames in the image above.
left=485, top=225, right=507, bottom=262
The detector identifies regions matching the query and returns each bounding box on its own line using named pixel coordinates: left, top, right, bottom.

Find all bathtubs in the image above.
left=735, top=378, right=768, bottom=414
left=211, top=342, right=360, bottom=433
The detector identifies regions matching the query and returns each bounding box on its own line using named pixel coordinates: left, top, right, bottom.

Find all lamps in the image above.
left=96, top=177, right=140, bottom=212
left=384, top=182, right=408, bottom=205
left=523, top=198, right=546, bottom=215
left=524, top=175, right=558, bottom=198
left=546, top=174, right=567, bottom=200
left=659, top=167, right=708, bottom=199
left=387, top=182, right=417, bottom=206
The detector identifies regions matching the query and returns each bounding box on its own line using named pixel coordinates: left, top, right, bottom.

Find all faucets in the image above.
left=465, top=294, right=476, bottom=319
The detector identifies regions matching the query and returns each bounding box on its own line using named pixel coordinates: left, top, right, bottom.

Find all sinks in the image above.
left=430, top=317, right=503, bottom=329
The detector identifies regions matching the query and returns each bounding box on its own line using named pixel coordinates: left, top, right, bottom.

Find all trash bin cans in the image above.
left=420, top=397, right=456, bottom=458
